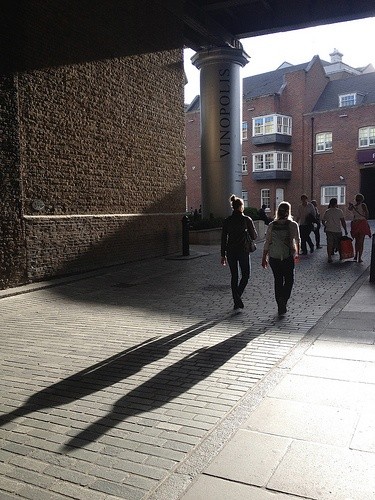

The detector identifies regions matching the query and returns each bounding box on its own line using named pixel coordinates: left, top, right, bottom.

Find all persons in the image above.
left=262, top=201, right=302, bottom=320
left=321, top=198, right=348, bottom=264
left=348, top=193, right=372, bottom=263
left=321, top=202, right=339, bottom=255
left=294, top=194, right=318, bottom=255
left=311, top=199, right=323, bottom=249
left=220, top=195, right=258, bottom=311
left=258, top=204, right=274, bottom=227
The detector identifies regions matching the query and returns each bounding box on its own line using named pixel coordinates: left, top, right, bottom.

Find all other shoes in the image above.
left=303, top=248, right=307, bottom=255
left=316, top=246, right=322, bottom=249
left=358, top=260, right=363, bottom=263
left=328, top=259, right=332, bottom=263
left=234, top=299, right=243, bottom=310
left=278, top=305, right=287, bottom=316
left=354, top=257, right=357, bottom=262
left=310, top=244, right=314, bottom=253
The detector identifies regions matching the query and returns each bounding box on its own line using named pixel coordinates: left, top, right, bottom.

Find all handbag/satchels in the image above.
left=245, top=217, right=256, bottom=252
left=339, top=235, right=354, bottom=260
